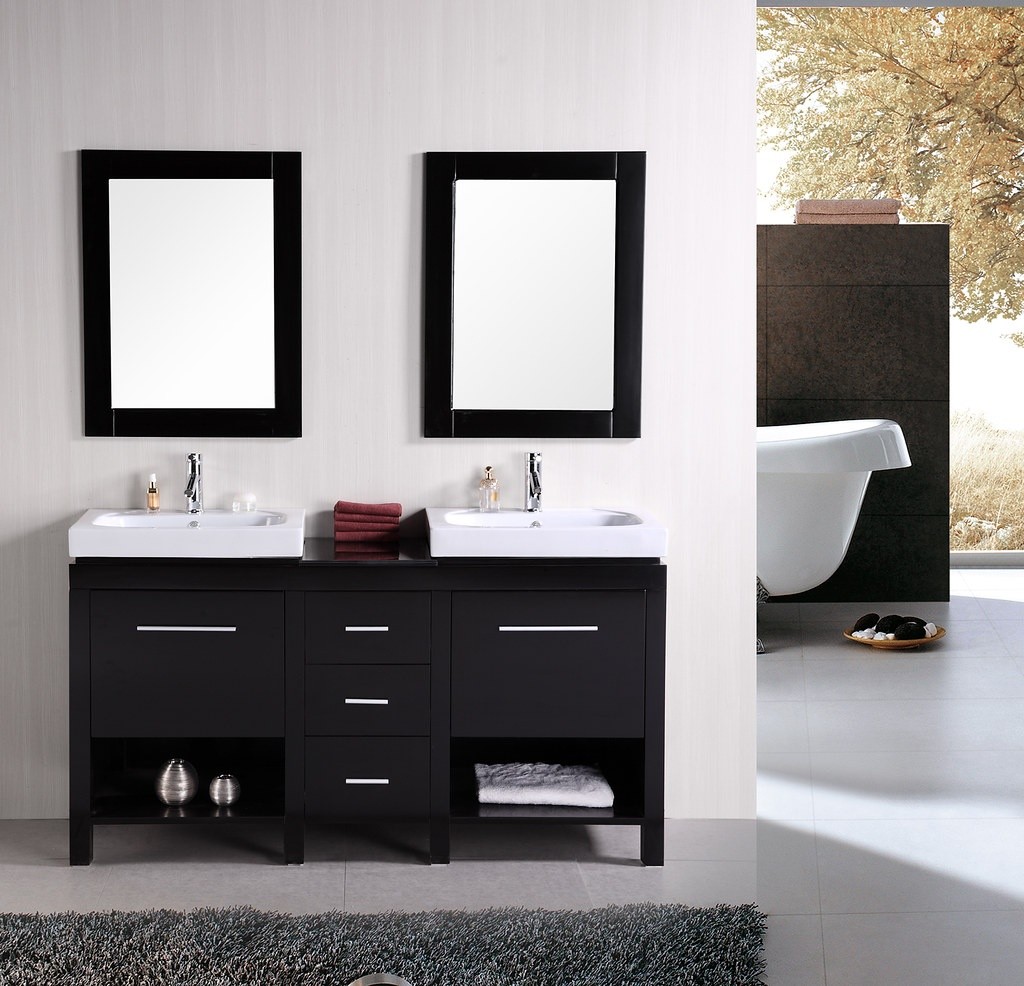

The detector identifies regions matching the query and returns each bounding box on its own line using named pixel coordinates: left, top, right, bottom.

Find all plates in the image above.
left=844, top=625, right=946, bottom=649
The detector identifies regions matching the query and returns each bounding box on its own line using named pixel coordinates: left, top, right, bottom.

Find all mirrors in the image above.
left=424, top=152, right=645, bottom=440
left=77, top=148, right=304, bottom=438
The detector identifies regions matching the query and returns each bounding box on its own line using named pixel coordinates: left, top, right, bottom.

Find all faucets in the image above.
left=523, top=452, right=542, bottom=513
left=183, top=452, right=205, bottom=515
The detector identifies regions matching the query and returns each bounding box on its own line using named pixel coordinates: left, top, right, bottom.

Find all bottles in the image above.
left=147, top=473, right=160, bottom=511
left=478, top=466, right=499, bottom=511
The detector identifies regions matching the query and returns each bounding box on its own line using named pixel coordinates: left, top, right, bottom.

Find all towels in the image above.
left=333, top=500, right=403, bottom=553
left=794, top=198, right=902, bottom=225
left=469, top=762, right=615, bottom=808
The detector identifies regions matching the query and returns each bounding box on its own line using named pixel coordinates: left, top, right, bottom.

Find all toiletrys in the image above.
left=478, top=465, right=500, bottom=513
left=144, top=473, right=161, bottom=513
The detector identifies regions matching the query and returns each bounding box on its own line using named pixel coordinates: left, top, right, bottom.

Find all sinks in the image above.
left=445, top=513, right=644, bottom=527
left=92, top=508, right=288, bottom=529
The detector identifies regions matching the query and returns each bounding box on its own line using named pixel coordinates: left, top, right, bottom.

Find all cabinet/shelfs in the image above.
left=758, top=223, right=951, bottom=603
left=67, top=554, right=665, bottom=868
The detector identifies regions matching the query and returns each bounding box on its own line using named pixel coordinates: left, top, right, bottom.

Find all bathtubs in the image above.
left=755, top=418, right=913, bottom=597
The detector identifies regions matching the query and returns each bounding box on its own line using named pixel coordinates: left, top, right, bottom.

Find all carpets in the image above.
left=1, top=901, right=766, bottom=985
